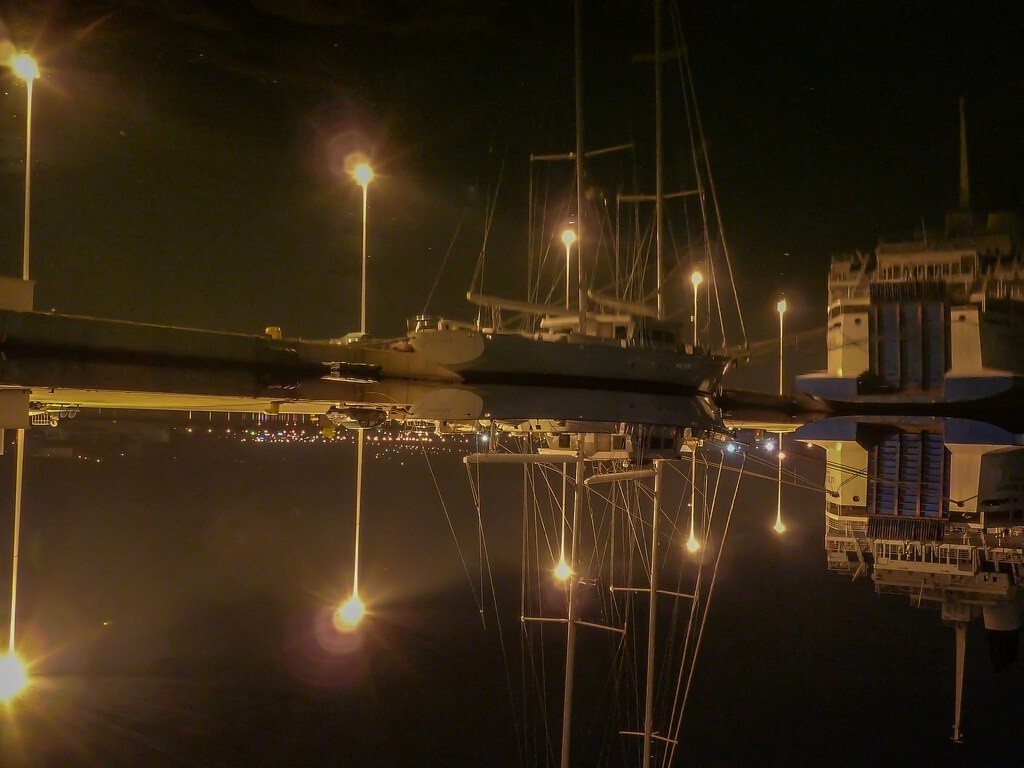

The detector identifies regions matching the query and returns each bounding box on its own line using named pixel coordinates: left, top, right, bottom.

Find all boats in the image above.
left=793, top=89, right=1024, bottom=425
left=784, top=431, right=1024, bottom=742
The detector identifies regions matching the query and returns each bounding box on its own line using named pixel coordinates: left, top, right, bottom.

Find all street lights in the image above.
left=691, top=270, right=703, bottom=348
left=550, top=459, right=575, bottom=585
left=562, top=229, right=576, bottom=310
left=772, top=432, right=788, bottom=535
left=4, top=46, right=43, bottom=279
left=685, top=448, right=700, bottom=554
left=335, top=429, right=368, bottom=626
left=350, top=158, right=375, bottom=331
left=1, top=426, right=31, bottom=706
left=777, top=301, right=786, bottom=395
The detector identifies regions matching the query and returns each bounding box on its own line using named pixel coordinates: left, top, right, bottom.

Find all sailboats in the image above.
left=351, top=389, right=762, bottom=768
left=355, top=0, right=756, bottom=388
